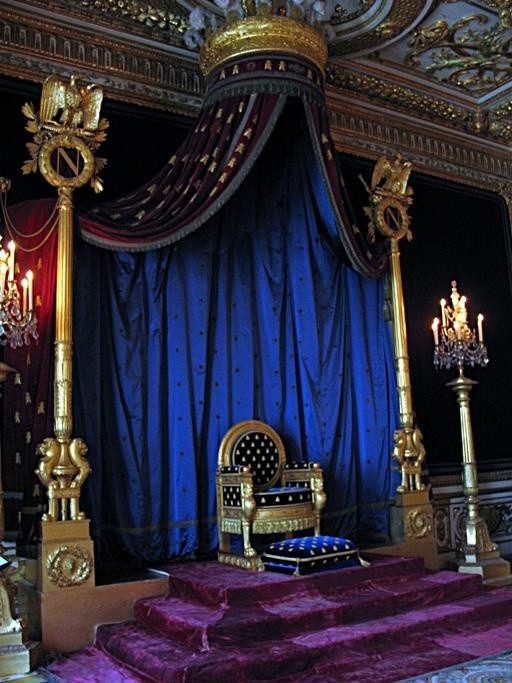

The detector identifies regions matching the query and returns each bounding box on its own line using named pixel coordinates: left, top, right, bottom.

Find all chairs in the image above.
left=214, top=418, right=328, bottom=574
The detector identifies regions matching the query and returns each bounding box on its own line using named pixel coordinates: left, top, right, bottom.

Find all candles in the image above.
left=435, top=315, right=442, bottom=336
left=438, top=298, right=452, bottom=329
left=429, top=322, right=442, bottom=339
left=6, top=236, right=14, bottom=283
left=478, top=315, right=487, bottom=343
left=21, top=278, right=31, bottom=312
left=457, top=294, right=470, bottom=318
left=26, top=269, right=35, bottom=312
left=0, top=248, right=12, bottom=296
